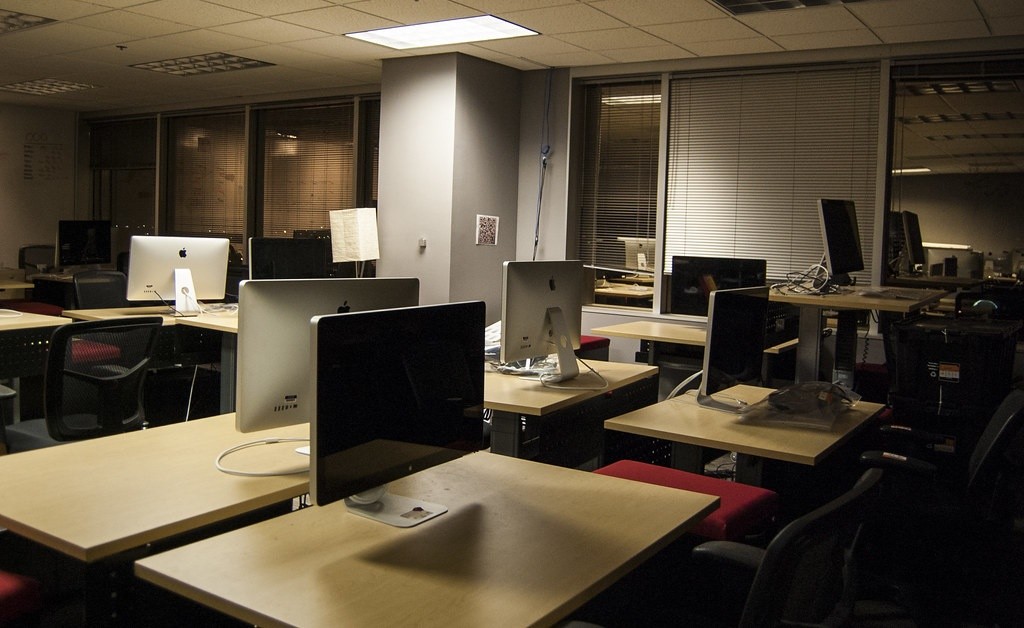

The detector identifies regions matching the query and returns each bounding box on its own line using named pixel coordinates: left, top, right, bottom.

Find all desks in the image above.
left=894, top=272, right=983, bottom=289
left=0, top=279, right=34, bottom=292
left=769, top=281, right=949, bottom=383
left=0, top=309, right=73, bottom=423
left=485, top=359, right=659, bottom=462
left=133, top=451, right=721, bottom=627
left=62, top=303, right=237, bottom=425
left=603, top=382, right=885, bottom=485
left=0, top=412, right=309, bottom=628
left=595, top=275, right=653, bottom=307
left=590, top=321, right=832, bottom=382
left=28, top=273, right=121, bottom=309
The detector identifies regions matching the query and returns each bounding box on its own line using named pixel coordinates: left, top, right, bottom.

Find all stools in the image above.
left=0, top=299, right=63, bottom=316
left=576, top=335, right=611, bottom=361
left=592, top=458, right=778, bottom=539
left=68, top=336, right=121, bottom=369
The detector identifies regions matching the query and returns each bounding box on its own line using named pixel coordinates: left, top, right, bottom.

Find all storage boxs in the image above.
left=887, top=314, right=1023, bottom=440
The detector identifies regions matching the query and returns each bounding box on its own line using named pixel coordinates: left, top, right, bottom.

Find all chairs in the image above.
left=952, top=270, right=1024, bottom=312
left=792, top=387, right=1024, bottom=614
left=559, top=467, right=886, bottom=628
left=0, top=270, right=164, bottom=455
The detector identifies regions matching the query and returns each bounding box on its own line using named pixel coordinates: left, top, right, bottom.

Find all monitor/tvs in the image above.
left=57, top=220, right=583, bottom=528
left=811, top=199, right=864, bottom=295
left=894, top=210, right=926, bottom=278
left=697, top=286, right=770, bottom=415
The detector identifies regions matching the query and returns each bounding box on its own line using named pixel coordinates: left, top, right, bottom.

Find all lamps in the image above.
left=330, top=208, right=380, bottom=278
